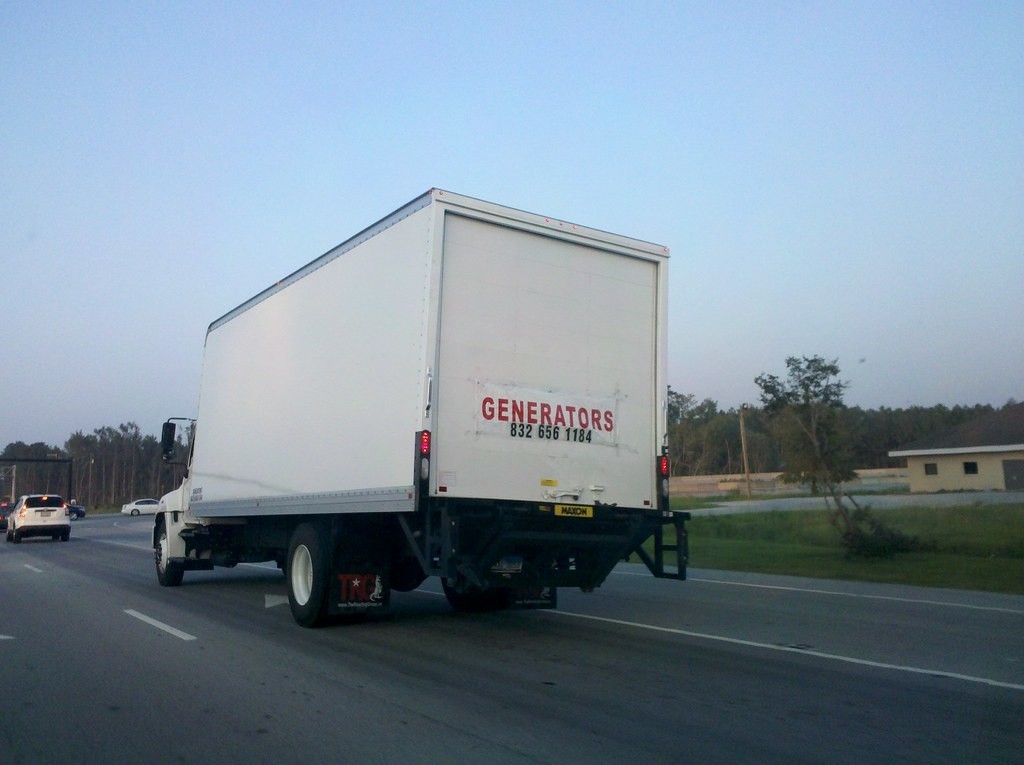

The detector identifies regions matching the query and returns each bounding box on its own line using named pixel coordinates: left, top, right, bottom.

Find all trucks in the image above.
left=153, top=188, right=690, bottom=630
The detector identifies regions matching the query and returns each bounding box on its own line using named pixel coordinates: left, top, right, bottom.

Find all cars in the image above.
left=121, top=498, right=160, bottom=516
left=0, top=494, right=86, bottom=543
